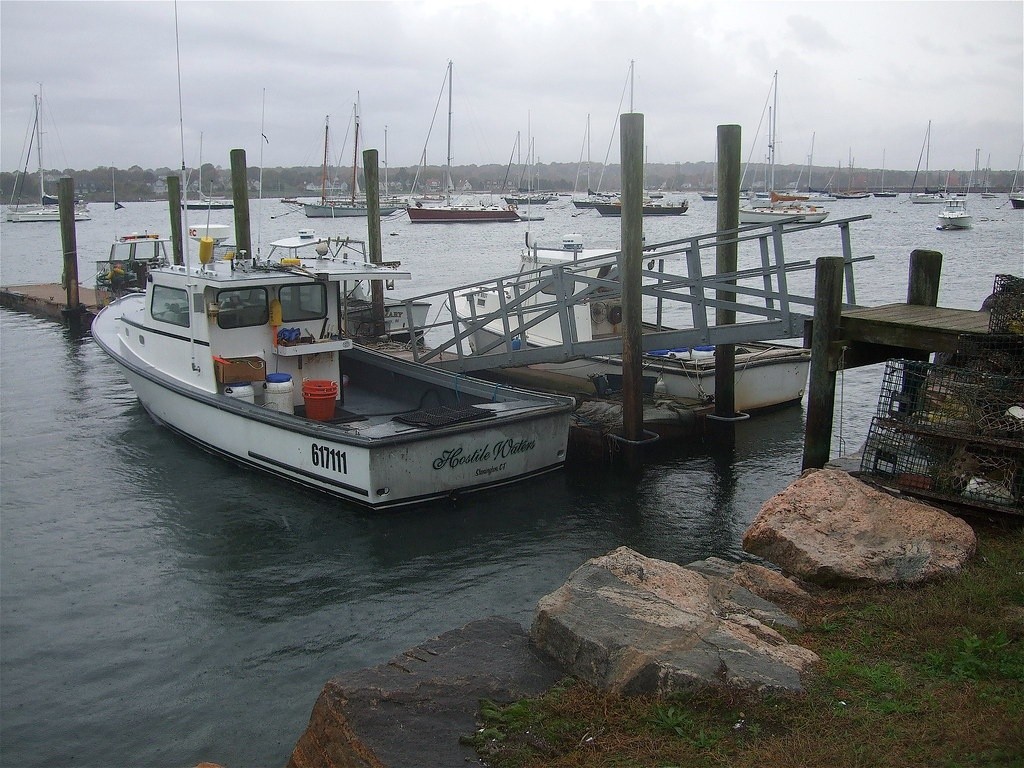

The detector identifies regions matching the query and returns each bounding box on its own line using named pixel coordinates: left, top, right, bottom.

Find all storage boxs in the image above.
left=214, top=356, right=266, bottom=384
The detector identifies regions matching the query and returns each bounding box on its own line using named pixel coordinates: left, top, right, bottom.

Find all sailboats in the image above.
left=700, top=68, right=897, bottom=224
left=1008, top=143, right=1024, bottom=209
left=570, top=58, right=690, bottom=217
left=908, top=120, right=995, bottom=205
left=500, top=110, right=572, bottom=205
left=181, top=129, right=234, bottom=210
left=6, top=81, right=91, bottom=223
left=282, top=90, right=408, bottom=217
left=403, top=60, right=522, bottom=224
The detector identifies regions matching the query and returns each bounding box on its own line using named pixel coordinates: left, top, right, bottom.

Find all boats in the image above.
left=937, top=199, right=973, bottom=230
left=90, top=179, right=577, bottom=514
left=444, top=233, right=811, bottom=419
left=265, top=229, right=432, bottom=341
left=96, top=232, right=173, bottom=295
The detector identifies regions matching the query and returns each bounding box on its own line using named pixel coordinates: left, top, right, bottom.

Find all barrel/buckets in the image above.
left=302, top=379, right=338, bottom=421
left=263, top=373, right=295, bottom=415
left=224, top=382, right=255, bottom=405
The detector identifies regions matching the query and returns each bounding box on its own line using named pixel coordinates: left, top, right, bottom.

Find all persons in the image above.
left=940, top=194, right=942, bottom=197
